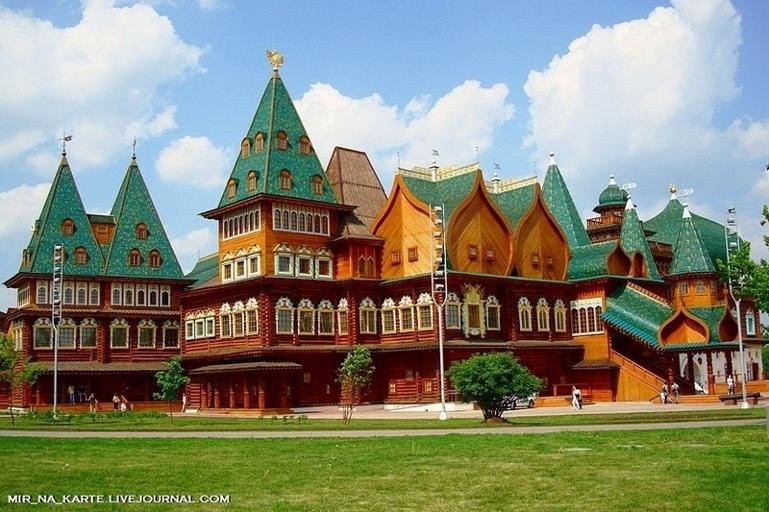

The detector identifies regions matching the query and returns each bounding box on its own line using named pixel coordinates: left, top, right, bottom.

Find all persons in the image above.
left=86, top=392, right=99, bottom=413
left=67, top=384, right=76, bottom=405
left=726, top=374, right=734, bottom=396
left=571, top=384, right=582, bottom=410
left=112, top=392, right=121, bottom=409
left=181, top=392, right=187, bottom=411
left=661, top=382, right=669, bottom=404
left=670, top=381, right=681, bottom=404
left=77, top=384, right=88, bottom=402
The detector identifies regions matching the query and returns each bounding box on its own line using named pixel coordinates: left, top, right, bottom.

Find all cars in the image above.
left=492, top=391, right=537, bottom=409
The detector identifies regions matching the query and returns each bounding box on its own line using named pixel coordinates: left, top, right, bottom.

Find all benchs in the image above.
left=719, top=392, right=760, bottom=406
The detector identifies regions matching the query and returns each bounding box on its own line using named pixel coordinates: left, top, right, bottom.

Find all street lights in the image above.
left=429, top=203, right=448, bottom=420
left=51, top=243, right=64, bottom=419
left=724, top=207, right=751, bottom=409
